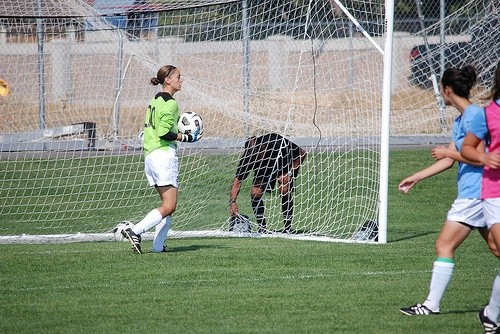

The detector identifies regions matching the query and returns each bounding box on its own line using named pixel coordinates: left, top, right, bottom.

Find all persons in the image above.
left=229, top=133, right=307, bottom=233
left=121, top=65, right=204, bottom=254
left=461, top=60, right=500, bottom=334
left=398, top=64, right=500, bottom=317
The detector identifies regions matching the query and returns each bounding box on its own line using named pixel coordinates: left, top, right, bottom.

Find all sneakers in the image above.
left=399, top=302, right=441, bottom=316
left=477, top=308, right=499, bottom=334
left=121, top=228, right=142, bottom=255
left=150, top=244, right=173, bottom=252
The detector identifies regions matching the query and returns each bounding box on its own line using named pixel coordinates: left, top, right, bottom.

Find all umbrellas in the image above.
left=0, top=0, right=118, bottom=130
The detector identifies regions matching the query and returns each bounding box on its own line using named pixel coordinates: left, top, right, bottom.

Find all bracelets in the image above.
left=229, top=198, right=237, bottom=206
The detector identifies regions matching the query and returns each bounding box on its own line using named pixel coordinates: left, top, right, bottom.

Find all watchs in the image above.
left=288, top=171, right=293, bottom=177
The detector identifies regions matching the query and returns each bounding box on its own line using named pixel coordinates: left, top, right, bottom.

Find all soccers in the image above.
left=178, top=111, right=203, bottom=135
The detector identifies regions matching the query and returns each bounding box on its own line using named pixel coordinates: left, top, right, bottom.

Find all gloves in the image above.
left=181, top=126, right=205, bottom=143
